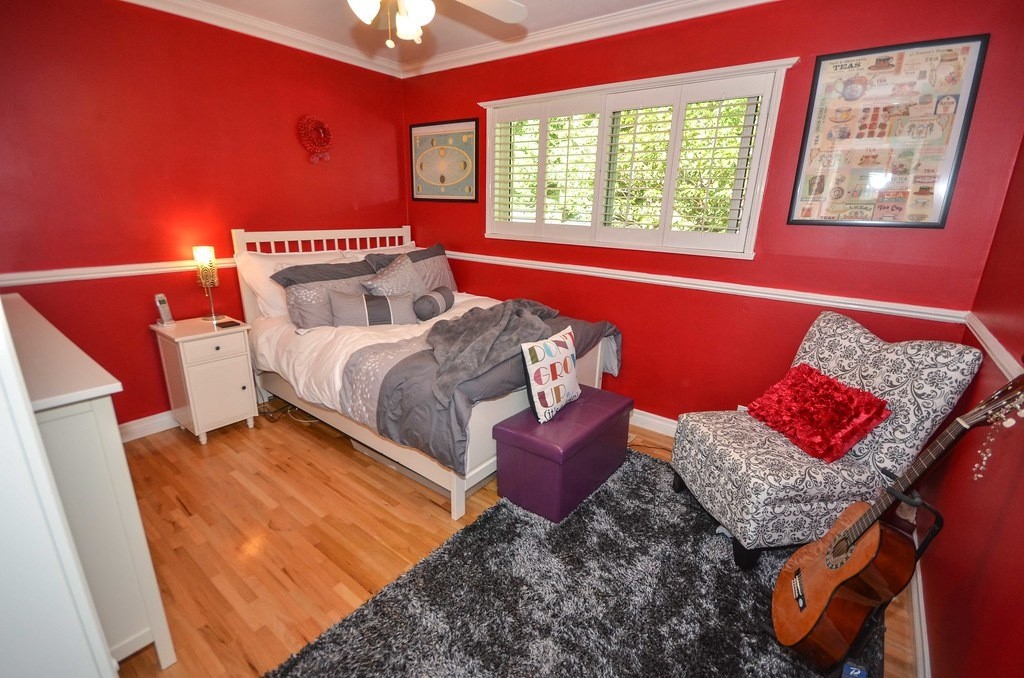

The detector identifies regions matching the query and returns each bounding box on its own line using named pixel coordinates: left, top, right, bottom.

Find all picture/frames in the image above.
left=785, top=31, right=992, bottom=228
left=408, top=117, right=479, bottom=204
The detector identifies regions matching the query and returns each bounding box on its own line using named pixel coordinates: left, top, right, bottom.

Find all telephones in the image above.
left=155, top=293, right=175, bottom=327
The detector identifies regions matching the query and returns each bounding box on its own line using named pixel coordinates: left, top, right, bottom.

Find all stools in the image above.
left=492, top=383, right=635, bottom=523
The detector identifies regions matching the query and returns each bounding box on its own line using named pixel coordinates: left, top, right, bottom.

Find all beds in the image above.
left=231, top=225, right=606, bottom=521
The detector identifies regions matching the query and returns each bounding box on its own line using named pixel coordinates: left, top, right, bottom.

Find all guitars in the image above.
left=771, top=373, right=1024, bottom=667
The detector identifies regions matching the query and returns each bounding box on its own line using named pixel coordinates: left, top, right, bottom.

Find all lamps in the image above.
left=347, top=0, right=436, bottom=49
left=193, top=244, right=226, bottom=321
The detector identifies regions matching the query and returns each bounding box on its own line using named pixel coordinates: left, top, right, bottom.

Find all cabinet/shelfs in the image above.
left=0, top=292, right=178, bottom=671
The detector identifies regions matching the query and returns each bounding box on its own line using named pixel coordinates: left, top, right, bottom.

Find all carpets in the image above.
left=261, top=449, right=887, bottom=678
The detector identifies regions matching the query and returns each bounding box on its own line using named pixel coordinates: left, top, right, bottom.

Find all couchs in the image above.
left=669, top=310, right=984, bottom=575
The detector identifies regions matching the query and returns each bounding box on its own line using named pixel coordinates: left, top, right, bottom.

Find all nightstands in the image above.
left=149, top=314, right=259, bottom=446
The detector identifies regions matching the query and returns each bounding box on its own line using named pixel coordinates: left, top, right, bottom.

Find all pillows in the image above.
left=522, top=324, right=582, bottom=424
left=269, top=259, right=377, bottom=331
left=232, top=248, right=347, bottom=320
left=414, top=285, right=455, bottom=321
left=365, top=241, right=459, bottom=295
left=327, top=288, right=419, bottom=327
left=342, top=241, right=416, bottom=262
left=745, top=363, right=893, bottom=463
left=361, top=252, right=427, bottom=296
